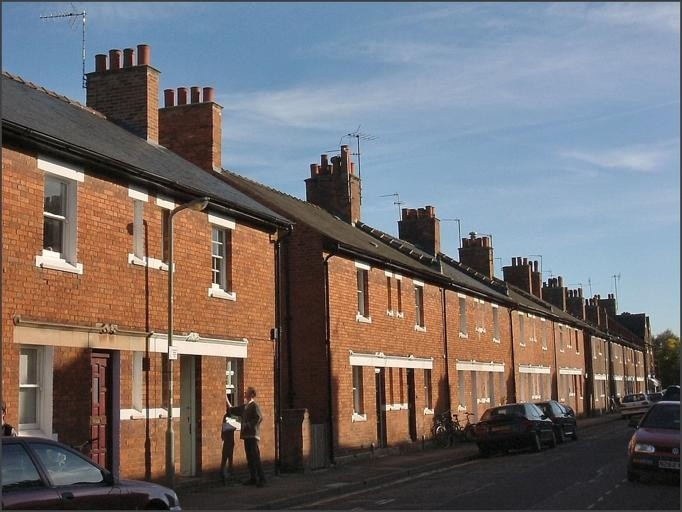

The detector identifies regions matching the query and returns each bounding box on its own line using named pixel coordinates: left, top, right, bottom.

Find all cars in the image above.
left=618, top=384, right=680, bottom=485
left=474, top=400, right=560, bottom=457
left=1, top=432, right=183, bottom=512
left=532, top=399, right=578, bottom=444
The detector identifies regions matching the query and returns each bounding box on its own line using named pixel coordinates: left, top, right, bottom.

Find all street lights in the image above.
left=163, top=193, right=213, bottom=487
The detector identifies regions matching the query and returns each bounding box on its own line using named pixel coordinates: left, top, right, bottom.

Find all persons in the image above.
left=222, top=385, right=267, bottom=487
left=605, top=391, right=615, bottom=414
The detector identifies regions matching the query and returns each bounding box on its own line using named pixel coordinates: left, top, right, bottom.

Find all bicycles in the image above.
left=432, top=411, right=478, bottom=449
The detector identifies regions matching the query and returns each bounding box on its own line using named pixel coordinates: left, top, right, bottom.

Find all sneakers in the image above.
left=257, top=479, right=268, bottom=488
left=243, top=478, right=257, bottom=486
